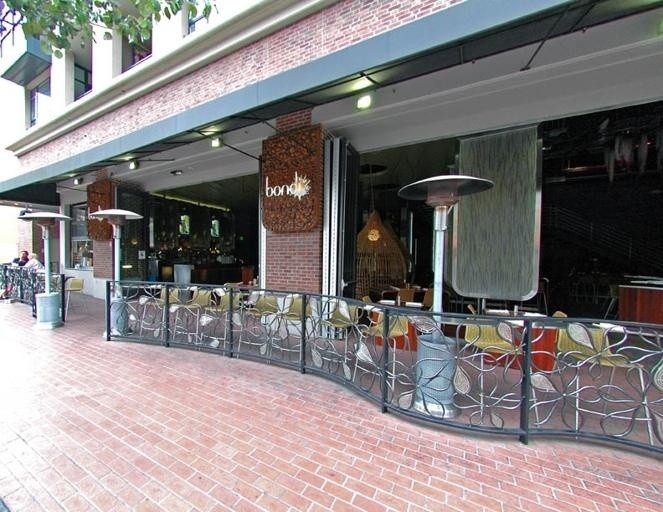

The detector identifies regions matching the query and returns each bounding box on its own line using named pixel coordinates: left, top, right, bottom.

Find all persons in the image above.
left=0, top=251, right=29, bottom=299
left=78, top=241, right=88, bottom=257
left=25, top=254, right=44, bottom=271
left=170, top=233, right=190, bottom=260
left=237, top=238, right=255, bottom=284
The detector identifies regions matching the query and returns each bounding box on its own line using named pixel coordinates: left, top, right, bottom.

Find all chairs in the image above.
left=62, top=277, right=87, bottom=321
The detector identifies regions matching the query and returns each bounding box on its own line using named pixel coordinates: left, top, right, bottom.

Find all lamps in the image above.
left=212, top=136, right=223, bottom=148
left=73, top=177, right=84, bottom=186
left=397, top=172, right=493, bottom=420
left=129, top=161, right=140, bottom=170
